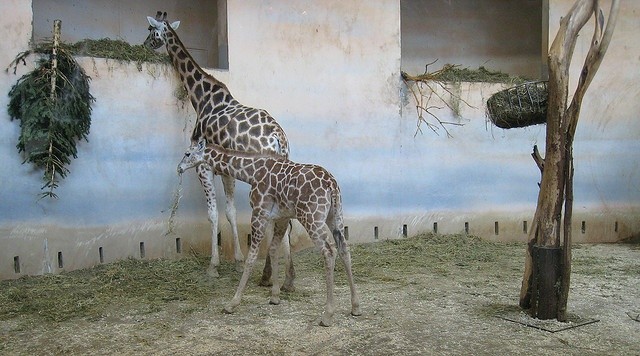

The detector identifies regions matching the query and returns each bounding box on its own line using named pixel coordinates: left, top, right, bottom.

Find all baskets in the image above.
left=487, top=80, right=549, bottom=129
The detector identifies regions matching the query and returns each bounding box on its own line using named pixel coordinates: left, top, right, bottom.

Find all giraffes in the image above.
left=176, top=137, right=363, bottom=329
left=142, top=10, right=299, bottom=294
